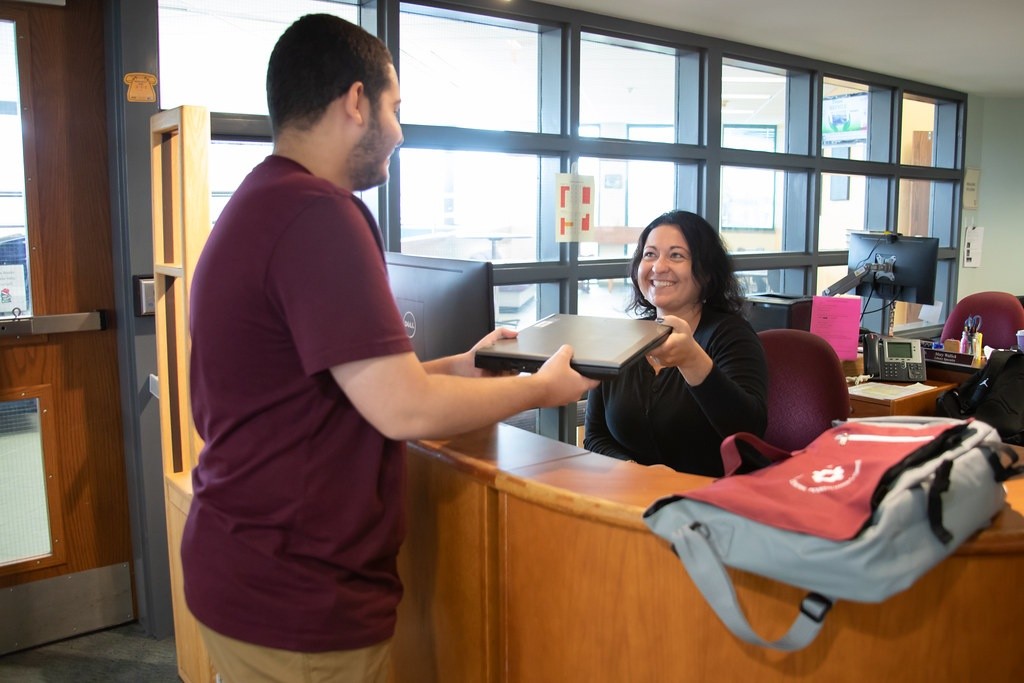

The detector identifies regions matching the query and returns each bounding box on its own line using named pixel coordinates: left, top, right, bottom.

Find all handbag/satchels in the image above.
left=644, top=416, right=1024, bottom=652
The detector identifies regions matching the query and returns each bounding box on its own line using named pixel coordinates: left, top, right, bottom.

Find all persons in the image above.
left=180, top=13, right=601, bottom=682
left=582, top=210, right=769, bottom=478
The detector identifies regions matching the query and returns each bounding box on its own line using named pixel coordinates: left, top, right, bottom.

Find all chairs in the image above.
left=747, top=328, right=851, bottom=465
left=940, top=291, right=1024, bottom=350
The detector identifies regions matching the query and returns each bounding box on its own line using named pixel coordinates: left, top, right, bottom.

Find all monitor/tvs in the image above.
left=821, top=234, right=938, bottom=306
left=382, top=251, right=494, bottom=362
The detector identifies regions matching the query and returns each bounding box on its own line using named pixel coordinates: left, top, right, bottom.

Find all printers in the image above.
left=742, top=292, right=813, bottom=333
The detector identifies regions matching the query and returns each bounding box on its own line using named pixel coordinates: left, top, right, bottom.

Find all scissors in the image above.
left=965, top=315, right=982, bottom=332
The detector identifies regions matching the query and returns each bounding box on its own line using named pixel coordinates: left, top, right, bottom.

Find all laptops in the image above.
left=475, top=313, right=672, bottom=380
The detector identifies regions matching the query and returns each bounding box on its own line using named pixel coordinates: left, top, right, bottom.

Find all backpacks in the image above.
left=934, top=349, right=1024, bottom=446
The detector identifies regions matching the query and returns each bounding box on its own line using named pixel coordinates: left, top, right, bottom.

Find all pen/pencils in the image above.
left=965, top=318, right=977, bottom=335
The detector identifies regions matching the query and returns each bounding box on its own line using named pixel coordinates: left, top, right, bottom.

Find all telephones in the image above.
left=863, top=333, right=927, bottom=383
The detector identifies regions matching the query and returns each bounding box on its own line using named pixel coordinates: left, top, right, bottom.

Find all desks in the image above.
left=846, top=379, right=959, bottom=420
